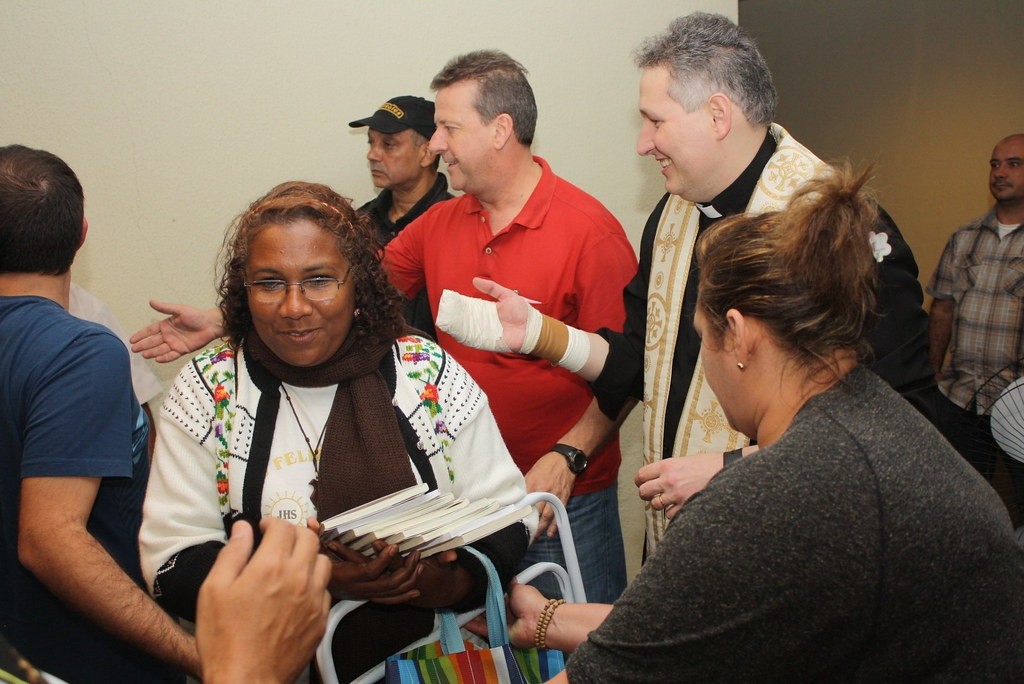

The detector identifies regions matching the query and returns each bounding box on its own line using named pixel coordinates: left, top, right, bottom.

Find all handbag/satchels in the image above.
left=384, top=544, right=565, bottom=684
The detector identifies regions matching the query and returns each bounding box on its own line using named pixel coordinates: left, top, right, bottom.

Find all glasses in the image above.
left=240, top=262, right=355, bottom=303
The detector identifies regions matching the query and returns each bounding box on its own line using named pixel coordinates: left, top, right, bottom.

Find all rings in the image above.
left=658, top=494, right=668, bottom=507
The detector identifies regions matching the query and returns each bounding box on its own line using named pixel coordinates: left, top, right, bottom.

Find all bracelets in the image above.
left=722, top=447, right=743, bottom=466
left=533, top=598, right=565, bottom=650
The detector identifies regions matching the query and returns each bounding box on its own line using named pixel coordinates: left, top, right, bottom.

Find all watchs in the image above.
left=550, top=443, right=588, bottom=475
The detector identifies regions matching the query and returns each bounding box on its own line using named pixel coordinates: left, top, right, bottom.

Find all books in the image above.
left=318, top=482, right=532, bottom=559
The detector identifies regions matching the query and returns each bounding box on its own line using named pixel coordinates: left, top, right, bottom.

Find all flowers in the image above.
left=871, top=231, right=891, bottom=261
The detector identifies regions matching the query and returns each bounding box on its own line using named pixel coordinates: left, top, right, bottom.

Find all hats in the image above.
left=349, top=94, right=437, bottom=141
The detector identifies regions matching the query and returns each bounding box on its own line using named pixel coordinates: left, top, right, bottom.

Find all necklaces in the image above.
left=277, top=383, right=330, bottom=511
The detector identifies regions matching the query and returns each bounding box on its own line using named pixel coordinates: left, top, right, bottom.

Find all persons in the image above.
left=130, top=49, right=641, bottom=663
left=435, top=10, right=928, bottom=566
left=349, top=94, right=456, bottom=344
left=924, top=131, right=1024, bottom=522
left=134, top=179, right=538, bottom=684
left=466, top=156, right=1024, bottom=684
left=0, top=143, right=204, bottom=684
left=195, top=517, right=332, bottom=684
